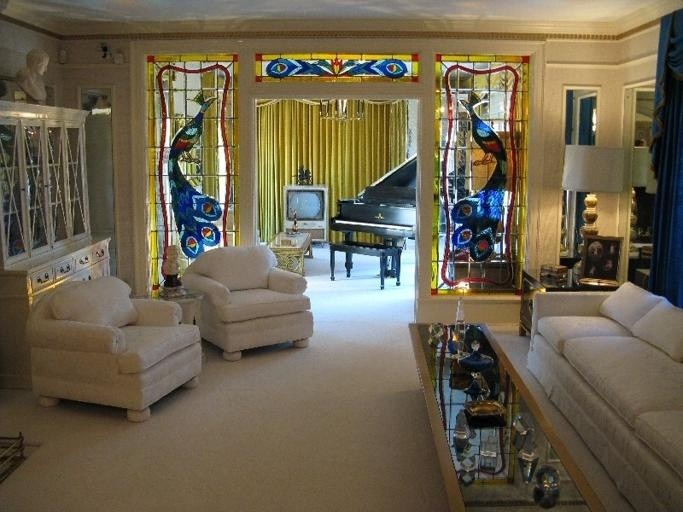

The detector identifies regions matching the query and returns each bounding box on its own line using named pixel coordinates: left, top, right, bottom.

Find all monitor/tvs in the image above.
left=283, top=185, right=327, bottom=230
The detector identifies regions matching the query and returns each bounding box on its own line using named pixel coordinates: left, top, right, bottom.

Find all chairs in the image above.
left=23, top=275, right=203, bottom=424
left=181, top=241, right=315, bottom=362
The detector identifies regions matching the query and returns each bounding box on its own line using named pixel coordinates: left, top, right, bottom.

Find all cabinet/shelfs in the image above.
left=519, top=268, right=564, bottom=336
left=0, top=99, right=117, bottom=394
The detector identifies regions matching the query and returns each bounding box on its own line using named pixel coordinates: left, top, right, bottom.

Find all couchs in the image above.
left=525, top=280, right=681, bottom=510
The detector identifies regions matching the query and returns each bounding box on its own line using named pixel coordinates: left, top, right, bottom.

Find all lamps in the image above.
left=562, top=142, right=650, bottom=273
left=317, top=99, right=366, bottom=125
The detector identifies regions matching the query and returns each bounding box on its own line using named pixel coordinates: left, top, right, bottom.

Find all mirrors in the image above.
left=620, top=78, right=665, bottom=281
left=74, top=83, right=121, bottom=276
left=556, top=82, right=599, bottom=266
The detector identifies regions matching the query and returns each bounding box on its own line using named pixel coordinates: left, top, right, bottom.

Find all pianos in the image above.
left=329, top=154, right=416, bottom=277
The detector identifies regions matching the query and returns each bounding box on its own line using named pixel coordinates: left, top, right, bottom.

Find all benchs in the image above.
left=328, top=239, right=402, bottom=289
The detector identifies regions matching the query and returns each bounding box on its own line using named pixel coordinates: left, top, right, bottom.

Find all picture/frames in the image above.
left=575, top=231, right=625, bottom=286
